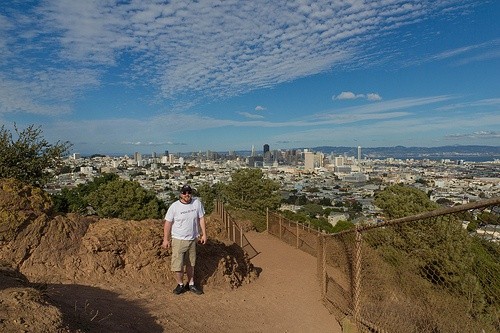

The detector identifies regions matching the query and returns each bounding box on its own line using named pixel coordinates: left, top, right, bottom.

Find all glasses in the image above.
left=180, top=191, right=192, bottom=195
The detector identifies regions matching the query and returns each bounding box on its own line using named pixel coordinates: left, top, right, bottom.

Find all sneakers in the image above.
left=173, top=283, right=186, bottom=295
left=185, top=282, right=203, bottom=295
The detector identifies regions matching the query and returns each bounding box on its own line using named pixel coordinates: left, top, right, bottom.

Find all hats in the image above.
left=181, top=185, right=192, bottom=191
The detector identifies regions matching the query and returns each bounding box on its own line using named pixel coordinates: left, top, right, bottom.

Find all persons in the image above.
left=163, top=184, right=207, bottom=294
